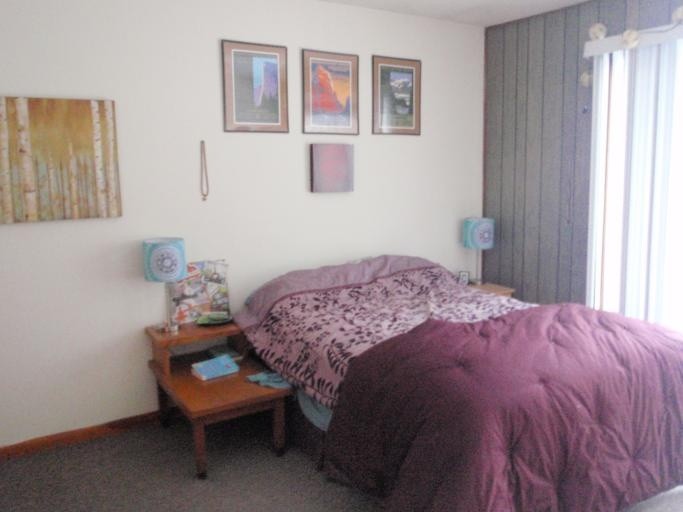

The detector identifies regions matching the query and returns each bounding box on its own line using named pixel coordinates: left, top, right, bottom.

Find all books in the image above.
left=191, top=344, right=244, bottom=363
left=188, top=354, right=241, bottom=381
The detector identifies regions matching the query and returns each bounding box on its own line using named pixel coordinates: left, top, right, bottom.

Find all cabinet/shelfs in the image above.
left=146, top=318, right=296, bottom=479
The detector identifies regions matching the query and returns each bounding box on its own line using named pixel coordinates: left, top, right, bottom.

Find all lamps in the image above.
left=142, top=236, right=187, bottom=334
left=462, top=216, right=496, bottom=286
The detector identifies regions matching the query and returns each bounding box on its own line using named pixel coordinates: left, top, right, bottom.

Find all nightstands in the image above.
left=470, top=282, right=515, bottom=298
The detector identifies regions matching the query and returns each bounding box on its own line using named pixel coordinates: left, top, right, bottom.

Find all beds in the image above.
left=231, top=255, right=683, bottom=512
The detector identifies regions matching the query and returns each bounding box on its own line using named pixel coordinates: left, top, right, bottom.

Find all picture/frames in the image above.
left=221, top=39, right=289, bottom=133
left=302, top=48, right=361, bottom=136
left=372, top=55, right=422, bottom=136
